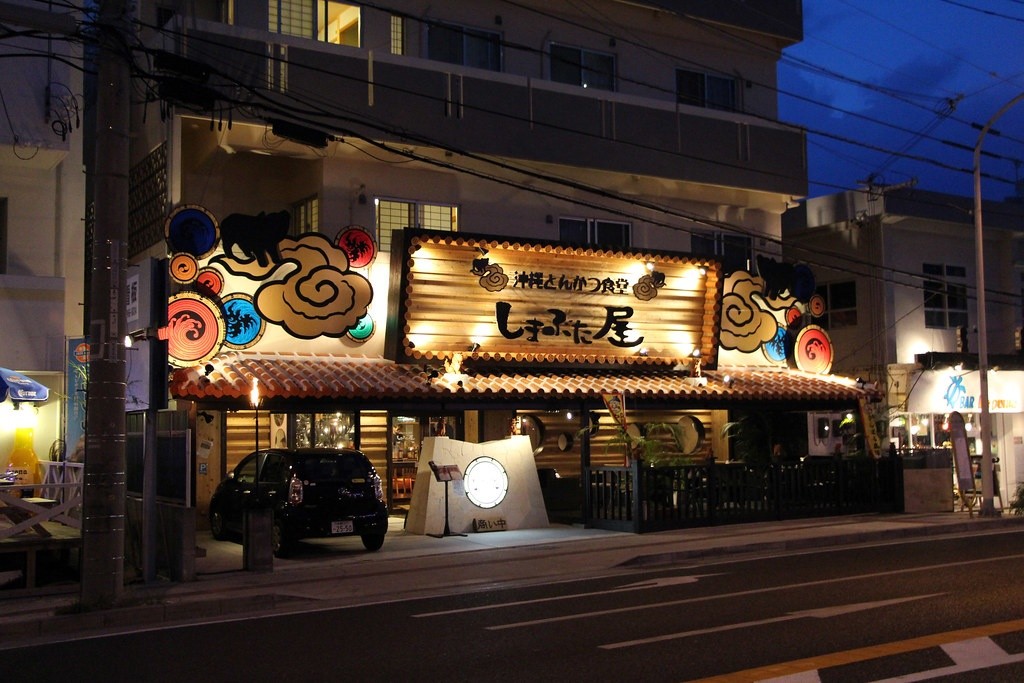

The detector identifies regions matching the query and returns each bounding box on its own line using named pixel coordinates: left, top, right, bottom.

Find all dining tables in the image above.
left=0, top=478, right=14, bottom=485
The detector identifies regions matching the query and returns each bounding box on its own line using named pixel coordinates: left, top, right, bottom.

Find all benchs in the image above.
left=20, top=497, right=57, bottom=506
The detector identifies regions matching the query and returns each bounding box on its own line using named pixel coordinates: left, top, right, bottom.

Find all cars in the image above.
left=207, top=447, right=390, bottom=550
